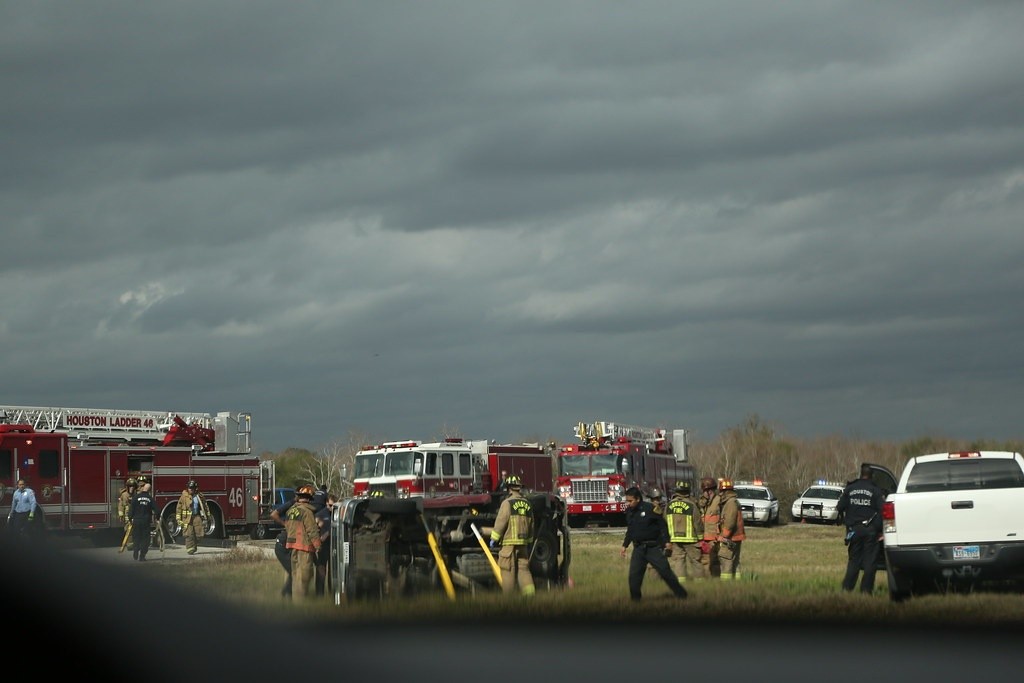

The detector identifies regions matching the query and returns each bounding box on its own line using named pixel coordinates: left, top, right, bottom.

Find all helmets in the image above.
left=676, top=481, right=691, bottom=491
left=861, top=466, right=874, bottom=475
left=505, top=476, right=522, bottom=486
left=716, top=476, right=735, bottom=489
left=296, top=487, right=316, bottom=504
left=649, top=488, right=662, bottom=498
left=124, top=477, right=137, bottom=487
left=699, top=477, right=713, bottom=491
left=137, top=475, right=148, bottom=483
left=186, top=480, right=197, bottom=488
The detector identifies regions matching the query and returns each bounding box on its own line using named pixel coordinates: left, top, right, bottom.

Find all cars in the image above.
left=331, top=498, right=570, bottom=602
left=732, top=481, right=780, bottom=526
left=791, top=480, right=846, bottom=525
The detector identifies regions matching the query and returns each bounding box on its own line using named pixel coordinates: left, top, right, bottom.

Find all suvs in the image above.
left=250, top=488, right=297, bottom=539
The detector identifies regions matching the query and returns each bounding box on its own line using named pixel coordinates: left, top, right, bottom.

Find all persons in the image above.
left=116, top=475, right=158, bottom=561
left=7, top=478, right=37, bottom=534
left=836, top=465, right=886, bottom=596
left=647, top=477, right=747, bottom=581
left=489, top=474, right=538, bottom=600
left=620, top=487, right=688, bottom=600
left=176, top=479, right=212, bottom=553
left=269, top=483, right=338, bottom=604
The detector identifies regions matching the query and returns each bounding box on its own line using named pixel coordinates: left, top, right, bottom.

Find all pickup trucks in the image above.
left=860, top=450, right=1023, bottom=601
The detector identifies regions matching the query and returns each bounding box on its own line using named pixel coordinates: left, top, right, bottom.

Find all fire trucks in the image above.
left=339, top=437, right=553, bottom=499
left=553, top=422, right=695, bottom=527
left=0, top=405, right=276, bottom=542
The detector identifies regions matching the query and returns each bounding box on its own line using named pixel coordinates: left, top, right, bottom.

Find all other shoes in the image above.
left=188, top=549, right=196, bottom=555
left=133, top=554, right=147, bottom=562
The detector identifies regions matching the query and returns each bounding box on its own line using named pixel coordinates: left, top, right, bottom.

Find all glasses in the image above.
left=325, top=504, right=334, bottom=508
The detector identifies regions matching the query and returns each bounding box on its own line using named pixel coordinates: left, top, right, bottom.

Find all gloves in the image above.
left=28, top=512, right=34, bottom=521
left=489, top=540, right=495, bottom=551
